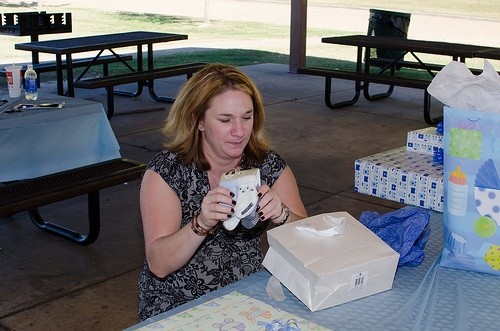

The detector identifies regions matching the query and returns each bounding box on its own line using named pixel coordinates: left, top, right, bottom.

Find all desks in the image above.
left=0, top=91, right=122, bottom=246
left=14, top=32, right=188, bottom=120
left=322, top=35, right=500, bottom=125
left=119, top=206, right=500, bottom=331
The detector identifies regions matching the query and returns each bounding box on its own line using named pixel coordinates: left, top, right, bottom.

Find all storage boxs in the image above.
left=354, top=126, right=446, bottom=213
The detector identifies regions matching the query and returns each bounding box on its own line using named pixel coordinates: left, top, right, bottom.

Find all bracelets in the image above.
left=270, top=202, right=289, bottom=227
left=190, top=215, right=213, bottom=236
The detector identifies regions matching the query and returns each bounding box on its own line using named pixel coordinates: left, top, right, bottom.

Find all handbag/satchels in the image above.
left=261, top=212, right=400, bottom=312
left=439, top=105, right=500, bottom=275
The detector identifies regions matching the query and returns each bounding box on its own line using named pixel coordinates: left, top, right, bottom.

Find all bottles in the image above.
left=24, top=64, right=38, bottom=100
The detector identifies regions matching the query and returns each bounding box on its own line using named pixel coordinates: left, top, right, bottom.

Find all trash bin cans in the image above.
left=369, top=8, right=411, bottom=71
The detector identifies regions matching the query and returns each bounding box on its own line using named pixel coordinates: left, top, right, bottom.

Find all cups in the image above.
left=5, top=65, right=21, bottom=98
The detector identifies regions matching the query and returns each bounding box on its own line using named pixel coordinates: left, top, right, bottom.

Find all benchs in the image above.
left=0, top=159, right=146, bottom=246
left=370, top=58, right=500, bottom=125
left=297, top=67, right=443, bottom=125
left=74, top=62, right=208, bottom=120
left=0, top=54, right=133, bottom=120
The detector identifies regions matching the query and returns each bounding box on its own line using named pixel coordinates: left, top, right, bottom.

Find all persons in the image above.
left=138, top=63, right=310, bottom=321
